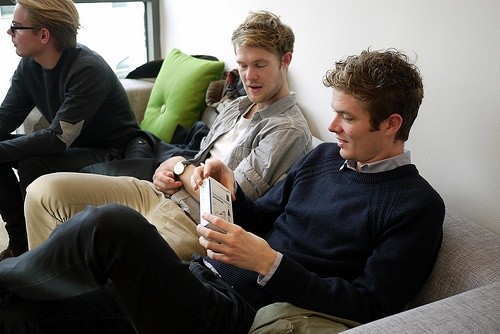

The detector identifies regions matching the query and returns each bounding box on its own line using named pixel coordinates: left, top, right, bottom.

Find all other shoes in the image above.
left=0, top=246, right=27, bottom=261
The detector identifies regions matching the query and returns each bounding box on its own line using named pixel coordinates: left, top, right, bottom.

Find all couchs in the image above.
left=22, top=78, right=500, bottom=334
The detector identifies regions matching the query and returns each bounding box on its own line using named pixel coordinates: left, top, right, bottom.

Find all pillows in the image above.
left=139, top=48, right=225, bottom=143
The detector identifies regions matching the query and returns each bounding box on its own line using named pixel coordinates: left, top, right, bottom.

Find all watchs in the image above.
left=173, top=160, right=191, bottom=179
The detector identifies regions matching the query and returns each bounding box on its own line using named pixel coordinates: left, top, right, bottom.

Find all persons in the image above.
left=0, top=0, right=138, bottom=259
left=24, top=13, right=313, bottom=263
left=0, top=51, right=445, bottom=334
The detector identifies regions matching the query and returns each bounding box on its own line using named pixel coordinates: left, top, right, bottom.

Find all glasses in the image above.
left=11, top=21, right=37, bottom=33
